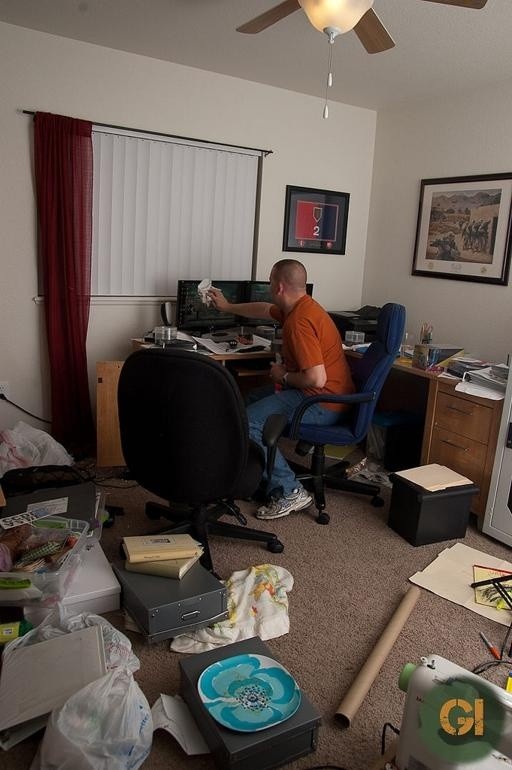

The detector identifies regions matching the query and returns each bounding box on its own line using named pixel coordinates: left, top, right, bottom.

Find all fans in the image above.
left=236, top=1, right=487, bottom=118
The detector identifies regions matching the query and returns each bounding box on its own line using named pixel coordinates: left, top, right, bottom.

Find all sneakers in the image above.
left=255, top=483, right=314, bottom=519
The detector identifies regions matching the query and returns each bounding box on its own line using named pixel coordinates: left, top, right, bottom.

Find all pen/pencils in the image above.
left=420, top=318, right=435, bottom=344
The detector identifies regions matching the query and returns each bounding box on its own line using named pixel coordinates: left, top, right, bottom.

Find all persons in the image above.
left=204, top=258, right=354, bottom=520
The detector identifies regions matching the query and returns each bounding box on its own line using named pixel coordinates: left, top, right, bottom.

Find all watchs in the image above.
left=281, top=370, right=289, bottom=388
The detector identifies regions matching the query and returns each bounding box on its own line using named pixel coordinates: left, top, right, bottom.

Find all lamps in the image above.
left=296, top=0, right=372, bottom=46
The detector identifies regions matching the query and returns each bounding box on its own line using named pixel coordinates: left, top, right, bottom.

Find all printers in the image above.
left=327, top=305, right=381, bottom=348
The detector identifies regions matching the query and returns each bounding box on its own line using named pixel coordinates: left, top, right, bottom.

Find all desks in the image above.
left=129, top=331, right=281, bottom=380
left=345, top=345, right=504, bottom=531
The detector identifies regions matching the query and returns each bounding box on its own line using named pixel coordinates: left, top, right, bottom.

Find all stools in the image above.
left=386, top=462, right=478, bottom=547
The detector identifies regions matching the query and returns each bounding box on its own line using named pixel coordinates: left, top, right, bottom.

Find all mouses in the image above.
left=227, top=339, right=238, bottom=349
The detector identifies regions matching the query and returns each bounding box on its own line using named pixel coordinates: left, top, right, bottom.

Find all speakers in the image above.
left=160, top=302, right=171, bottom=324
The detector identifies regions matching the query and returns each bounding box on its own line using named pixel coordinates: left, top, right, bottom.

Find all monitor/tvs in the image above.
left=246, top=281, right=313, bottom=345
left=176, top=280, right=249, bottom=341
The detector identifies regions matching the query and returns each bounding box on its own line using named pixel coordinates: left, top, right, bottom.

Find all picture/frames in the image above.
left=410, top=173, right=510, bottom=286
left=279, top=185, right=351, bottom=259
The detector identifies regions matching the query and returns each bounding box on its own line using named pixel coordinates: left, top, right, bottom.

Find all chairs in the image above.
left=260, top=303, right=405, bottom=527
left=118, top=348, right=285, bottom=582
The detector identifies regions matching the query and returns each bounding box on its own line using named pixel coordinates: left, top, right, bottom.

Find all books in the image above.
left=124, top=545, right=206, bottom=580
left=119, top=530, right=200, bottom=561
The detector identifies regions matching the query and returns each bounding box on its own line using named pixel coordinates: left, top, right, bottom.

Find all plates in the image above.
left=197, top=654, right=301, bottom=734
